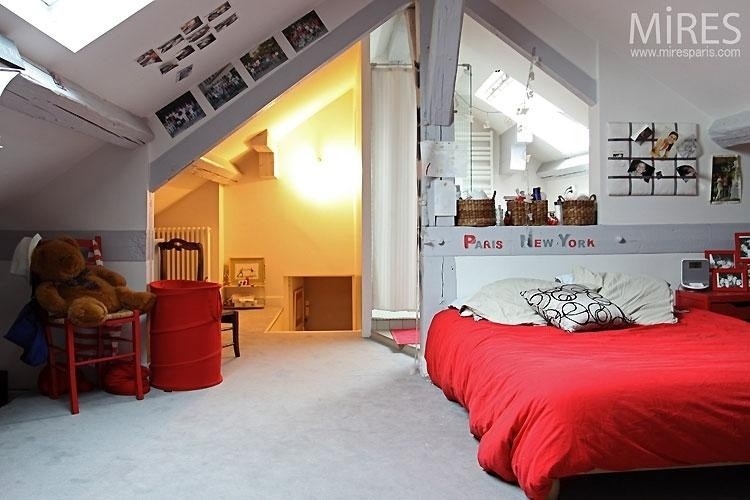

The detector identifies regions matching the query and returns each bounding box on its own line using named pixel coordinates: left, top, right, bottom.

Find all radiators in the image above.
left=155, top=225, right=211, bottom=282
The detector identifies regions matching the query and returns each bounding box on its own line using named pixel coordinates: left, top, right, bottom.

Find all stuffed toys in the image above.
left=30, top=234, right=158, bottom=326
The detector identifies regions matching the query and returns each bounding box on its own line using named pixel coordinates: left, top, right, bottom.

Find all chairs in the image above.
left=32, top=235, right=241, bottom=415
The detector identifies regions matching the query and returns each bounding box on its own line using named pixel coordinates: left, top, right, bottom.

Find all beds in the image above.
left=425, top=255, right=750, bottom=499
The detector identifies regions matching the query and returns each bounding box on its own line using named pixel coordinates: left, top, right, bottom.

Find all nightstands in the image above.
left=675, top=289, right=750, bottom=324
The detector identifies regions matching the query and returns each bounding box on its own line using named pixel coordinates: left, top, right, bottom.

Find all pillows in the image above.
left=462, top=264, right=678, bottom=334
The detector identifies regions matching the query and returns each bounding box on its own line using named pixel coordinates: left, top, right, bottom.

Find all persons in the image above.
left=166, top=98, right=202, bottom=134
left=290, top=14, right=322, bottom=45
left=628, top=161, right=649, bottom=177
left=711, top=238, right=750, bottom=288
left=244, top=41, right=283, bottom=78
left=650, top=130, right=679, bottom=158
left=203, top=68, right=244, bottom=105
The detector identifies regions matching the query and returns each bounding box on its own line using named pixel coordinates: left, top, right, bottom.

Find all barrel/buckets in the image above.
left=149, top=279, right=224, bottom=391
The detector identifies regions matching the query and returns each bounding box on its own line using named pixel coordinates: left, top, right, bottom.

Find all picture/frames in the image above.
left=704, top=233, right=750, bottom=292
left=229, top=257, right=265, bottom=284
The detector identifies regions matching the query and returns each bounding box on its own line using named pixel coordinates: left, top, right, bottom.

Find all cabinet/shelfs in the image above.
left=224, top=284, right=266, bottom=310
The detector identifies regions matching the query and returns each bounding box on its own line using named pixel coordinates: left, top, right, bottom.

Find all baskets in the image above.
left=507, top=200, right=547, bottom=226
left=558, top=194, right=596, bottom=225
left=456, top=191, right=496, bottom=227
